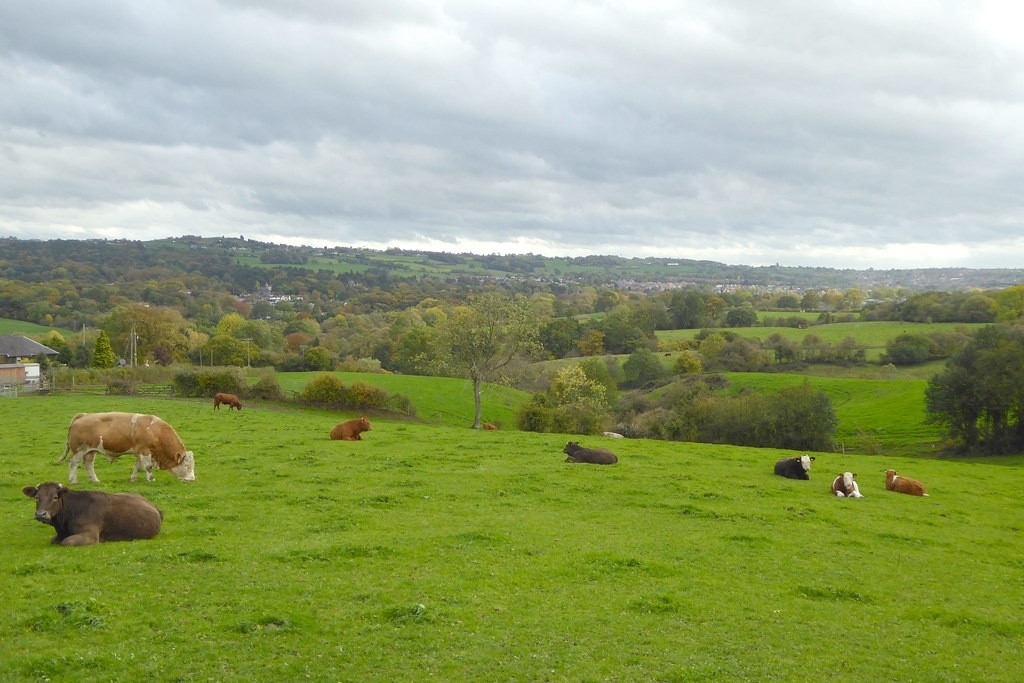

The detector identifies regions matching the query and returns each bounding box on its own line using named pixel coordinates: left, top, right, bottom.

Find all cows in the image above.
left=665, top=353, right=671, bottom=357
left=773, top=454, right=816, bottom=480
left=563, top=442, right=618, bottom=464
left=330, top=416, right=373, bottom=441
left=59, top=411, right=195, bottom=485
left=831, top=472, right=863, bottom=498
left=214, top=393, right=242, bottom=411
left=23, top=481, right=164, bottom=546
left=483, top=424, right=496, bottom=430
left=885, top=469, right=925, bottom=496
